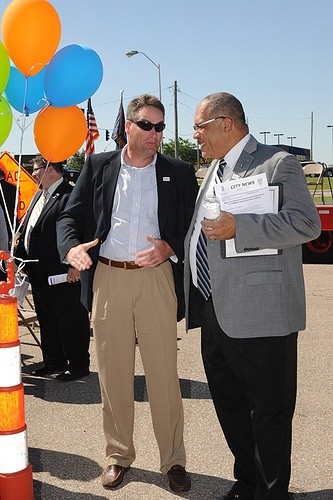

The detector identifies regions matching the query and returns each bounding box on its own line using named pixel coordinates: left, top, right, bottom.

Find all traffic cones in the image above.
left=0, top=295, right=34, bottom=500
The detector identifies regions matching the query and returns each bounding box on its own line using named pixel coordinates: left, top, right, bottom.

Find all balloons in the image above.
left=1, top=0, right=102, bottom=166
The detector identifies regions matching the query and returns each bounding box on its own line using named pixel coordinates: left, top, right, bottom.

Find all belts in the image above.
left=97, top=256, right=144, bottom=270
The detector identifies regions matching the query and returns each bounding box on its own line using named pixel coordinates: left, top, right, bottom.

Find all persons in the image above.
left=55, top=95, right=201, bottom=491
left=135, top=92, right=321, bottom=500
left=0, top=158, right=91, bottom=381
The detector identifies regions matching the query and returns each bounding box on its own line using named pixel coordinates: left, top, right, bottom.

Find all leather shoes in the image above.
left=167, top=465, right=191, bottom=492
left=101, top=465, right=130, bottom=490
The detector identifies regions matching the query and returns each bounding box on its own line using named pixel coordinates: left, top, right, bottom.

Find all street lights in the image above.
left=125, top=50, right=164, bottom=155
left=273, top=133, right=283, bottom=147
left=287, top=136, right=296, bottom=147
left=260, top=131, right=270, bottom=144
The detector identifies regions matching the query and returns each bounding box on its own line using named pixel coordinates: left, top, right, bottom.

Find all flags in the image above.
left=82, top=97, right=127, bottom=161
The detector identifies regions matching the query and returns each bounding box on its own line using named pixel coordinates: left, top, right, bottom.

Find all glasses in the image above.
left=193, top=116, right=233, bottom=130
left=129, top=119, right=165, bottom=132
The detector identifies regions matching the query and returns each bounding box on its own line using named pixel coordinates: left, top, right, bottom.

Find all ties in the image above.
left=195, top=157, right=227, bottom=302
left=27, top=189, right=49, bottom=251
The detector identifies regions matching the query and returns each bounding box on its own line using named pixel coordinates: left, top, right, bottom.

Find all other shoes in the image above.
left=56, top=366, right=90, bottom=381
left=216, top=481, right=241, bottom=500
left=32, top=364, right=68, bottom=376
